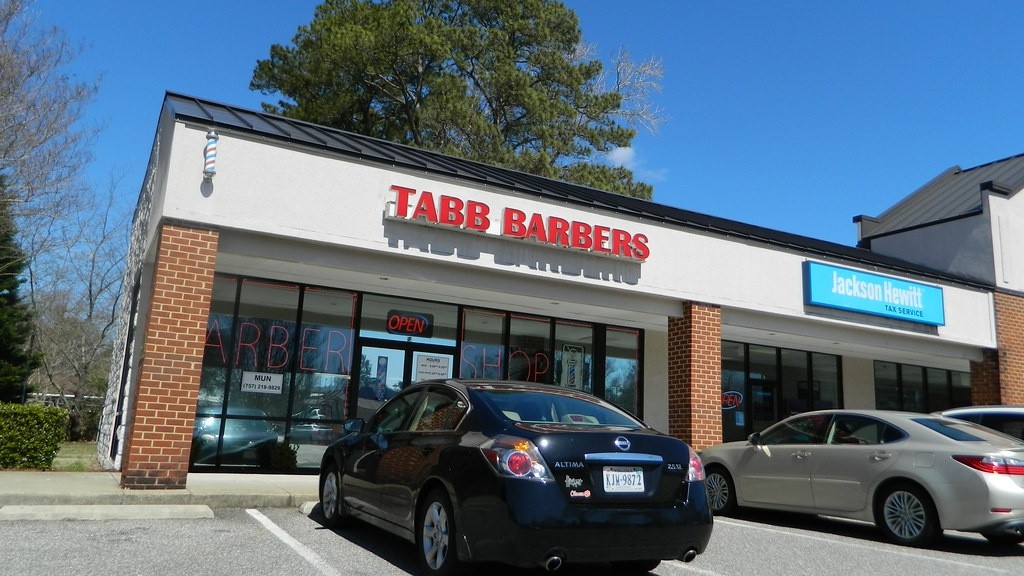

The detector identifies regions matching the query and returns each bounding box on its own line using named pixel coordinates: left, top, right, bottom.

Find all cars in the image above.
left=191, top=407, right=335, bottom=474
left=698, top=409, right=1024, bottom=549
left=941, top=404, right=1024, bottom=440
left=319, top=377, right=714, bottom=576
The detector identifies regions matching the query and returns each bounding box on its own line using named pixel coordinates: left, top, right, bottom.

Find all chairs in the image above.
left=502, top=410, right=521, bottom=420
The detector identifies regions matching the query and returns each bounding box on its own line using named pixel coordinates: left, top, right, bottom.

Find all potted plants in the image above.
left=261, top=440, right=296, bottom=470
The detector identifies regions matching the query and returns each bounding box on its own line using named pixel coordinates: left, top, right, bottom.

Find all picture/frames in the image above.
left=797, top=380, right=820, bottom=400
